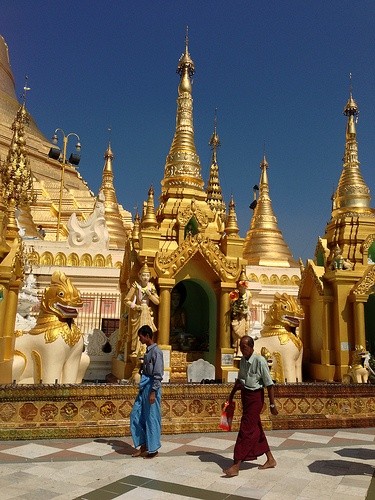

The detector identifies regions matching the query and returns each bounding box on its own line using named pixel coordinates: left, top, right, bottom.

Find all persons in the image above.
left=124, top=265, right=160, bottom=384
left=224, top=335, right=279, bottom=476
left=37, top=225, right=46, bottom=238
left=230, top=272, right=252, bottom=357
left=130, top=325, right=164, bottom=459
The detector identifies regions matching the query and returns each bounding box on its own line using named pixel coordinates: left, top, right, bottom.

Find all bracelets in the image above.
left=270, top=404, right=276, bottom=408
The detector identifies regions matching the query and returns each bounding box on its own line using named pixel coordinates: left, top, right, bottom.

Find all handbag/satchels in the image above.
left=218, top=400, right=236, bottom=431
